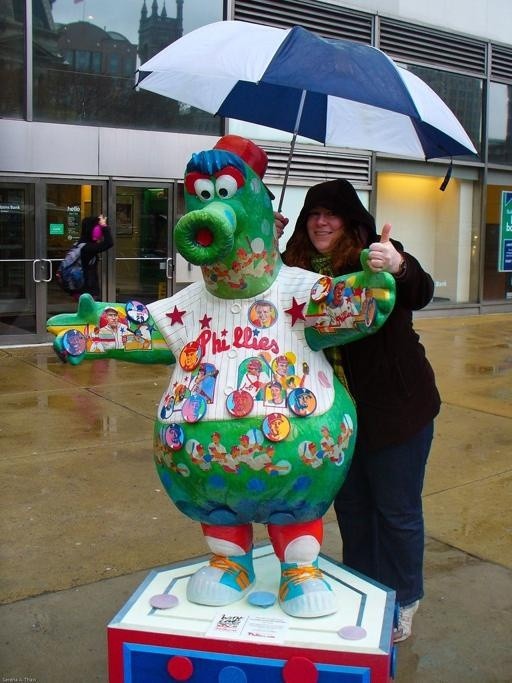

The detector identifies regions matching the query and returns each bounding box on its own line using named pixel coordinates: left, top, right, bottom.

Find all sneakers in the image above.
left=391, top=599, right=420, bottom=645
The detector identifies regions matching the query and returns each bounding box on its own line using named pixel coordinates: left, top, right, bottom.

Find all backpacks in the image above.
left=55, top=240, right=95, bottom=294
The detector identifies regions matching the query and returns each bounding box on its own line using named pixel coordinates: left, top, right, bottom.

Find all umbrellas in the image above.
left=129, top=17, right=481, bottom=225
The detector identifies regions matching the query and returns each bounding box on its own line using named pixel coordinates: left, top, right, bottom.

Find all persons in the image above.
left=52, top=213, right=113, bottom=364
left=270, top=178, right=442, bottom=644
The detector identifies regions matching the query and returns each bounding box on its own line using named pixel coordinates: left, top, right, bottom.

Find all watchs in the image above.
left=392, top=258, right=406, bottom=277
